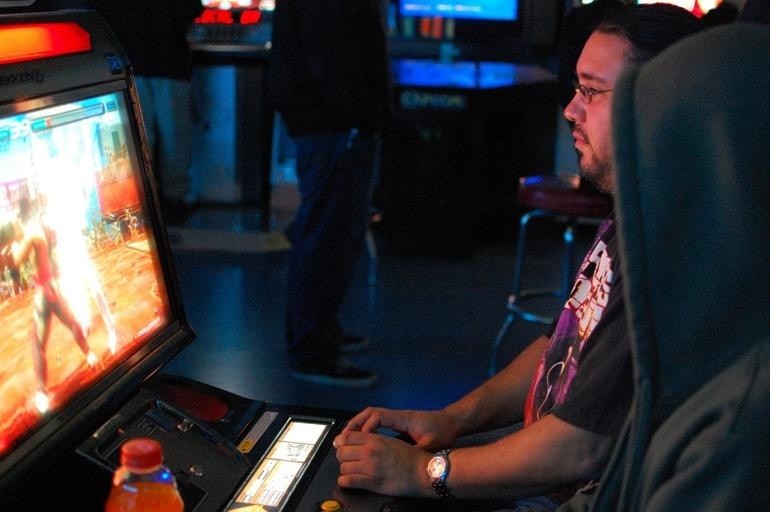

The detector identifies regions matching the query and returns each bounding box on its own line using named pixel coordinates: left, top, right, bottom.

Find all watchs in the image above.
left=421, top=445, right=467, bottom=500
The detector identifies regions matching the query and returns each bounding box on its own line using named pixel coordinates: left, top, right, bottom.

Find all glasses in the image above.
left=570, top=76, right=614, bottom=106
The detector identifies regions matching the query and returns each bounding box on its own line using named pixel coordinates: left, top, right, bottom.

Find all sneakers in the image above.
left=286, top=328, right=378, bottom=388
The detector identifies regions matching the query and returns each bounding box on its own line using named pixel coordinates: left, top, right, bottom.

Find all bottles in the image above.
left=104, top=438, right=186, bottom=512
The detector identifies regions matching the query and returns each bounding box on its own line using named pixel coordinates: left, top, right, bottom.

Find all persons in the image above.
left=333, top=7, right=710, bottom=504
left=1, top=144, right=144, bottom=412
left=271, top=5, right=390, bottom=394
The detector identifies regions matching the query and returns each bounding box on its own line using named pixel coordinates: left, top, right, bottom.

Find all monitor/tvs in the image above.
left=399, top=0, right=522, bottom=23
left=198, top=0, right=277, bottom=16
left=0, top=8, right=196, bottom=499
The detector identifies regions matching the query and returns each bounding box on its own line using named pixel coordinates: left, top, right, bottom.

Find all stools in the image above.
left=488, top=172, right=610, bottom=376
